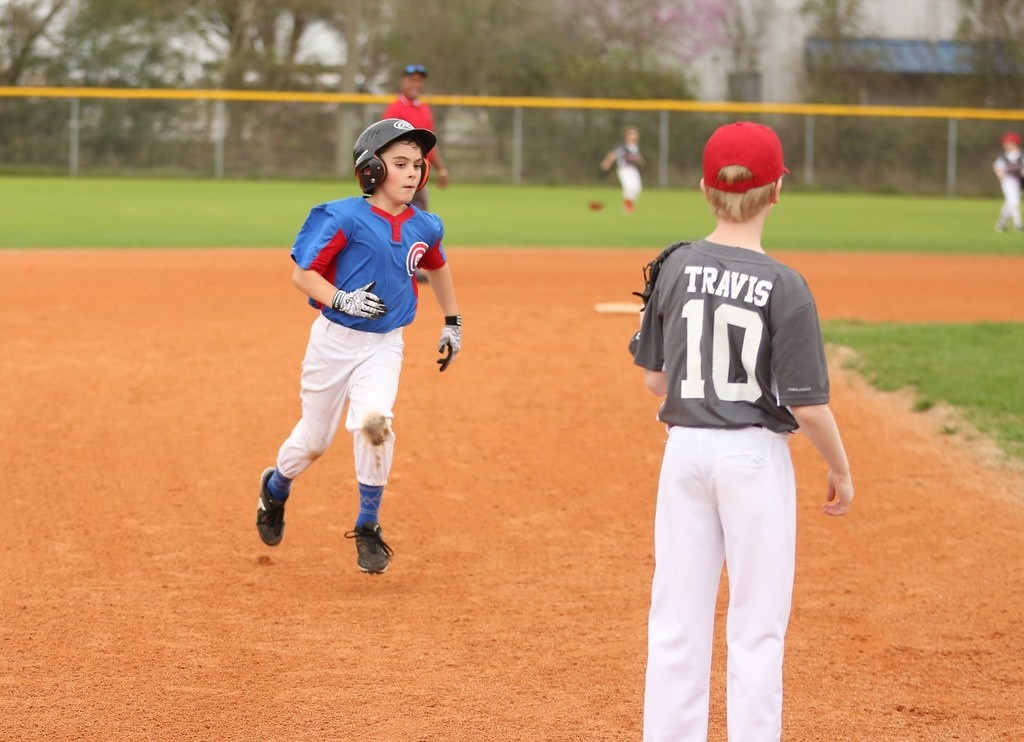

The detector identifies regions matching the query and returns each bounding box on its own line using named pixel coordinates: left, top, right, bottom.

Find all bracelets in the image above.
left=438, top=169, right=449, bottom=176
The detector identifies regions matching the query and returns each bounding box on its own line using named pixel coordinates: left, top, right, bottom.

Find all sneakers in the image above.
left=344, top=522, right=395, bottom=574
left=255, top=467, right=290, bottom=546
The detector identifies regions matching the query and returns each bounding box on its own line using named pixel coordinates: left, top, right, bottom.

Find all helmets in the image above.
left=351, top=116, right=437, bottom=193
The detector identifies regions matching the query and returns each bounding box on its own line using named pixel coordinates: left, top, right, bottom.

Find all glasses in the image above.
left=404, top=65, right=425, bottom=74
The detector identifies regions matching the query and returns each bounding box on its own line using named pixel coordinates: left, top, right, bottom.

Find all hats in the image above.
left=701, top=121, right=790, bottom=193
left=1000, top=132, right=1021, bottom=145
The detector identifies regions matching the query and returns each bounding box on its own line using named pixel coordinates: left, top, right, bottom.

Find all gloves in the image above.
left=436, top=315, right=462, bottom=373
left=331, top=280, right=388, bottom=321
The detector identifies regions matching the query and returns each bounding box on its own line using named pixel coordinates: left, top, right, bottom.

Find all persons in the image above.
left=629, top=121, right=854, bottom=742
left=256, top=118, right=461, bottom=574
left=993, top=133, right=1024, bottom=233
left=381, top=64, right=449, bottom=283
left=600, top=128, right=645, bottom=213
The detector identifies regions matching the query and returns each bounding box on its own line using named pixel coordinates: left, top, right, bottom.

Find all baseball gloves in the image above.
left=632, top=241, right=696, bottom=312
